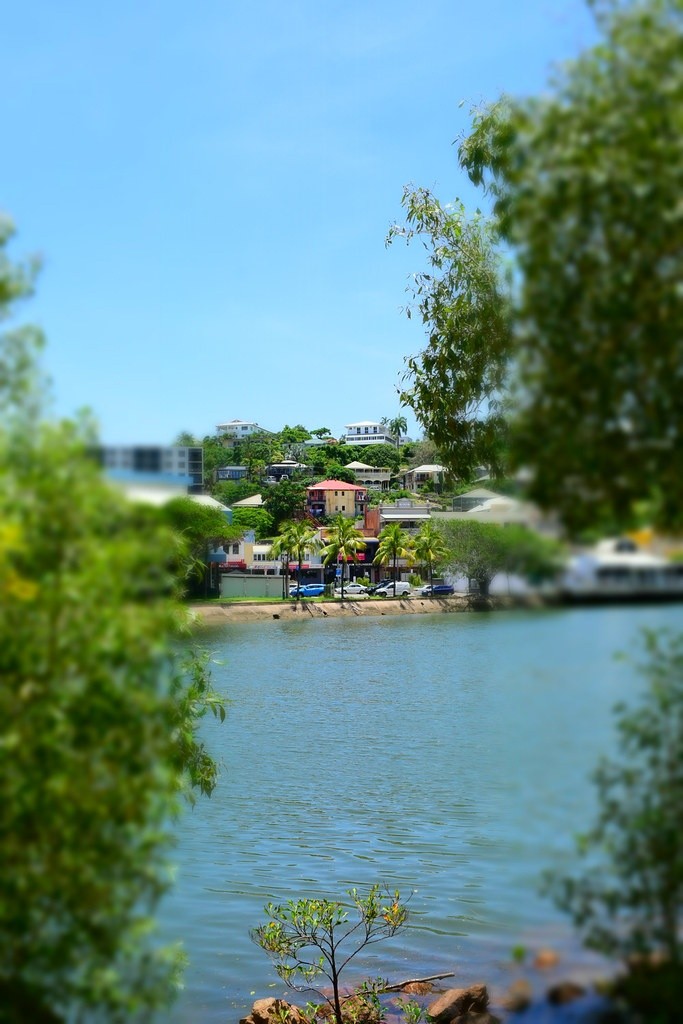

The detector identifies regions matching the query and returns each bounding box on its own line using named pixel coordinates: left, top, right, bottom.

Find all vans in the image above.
left=422, top=585, right=455, bottom=597
left=375, top=582, right=412, bottom=598
left=563, top=555, right=683, bottom=604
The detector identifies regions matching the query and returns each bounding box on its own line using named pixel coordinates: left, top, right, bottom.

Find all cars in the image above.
left=291, top=584, right=325, bottom=597
left=288, top=584, right=298, bottom=594
left=335, top=583, right=368, bottom=595
left=414, top=583, right=437, bottom=595
left=367, top=582, right=391, bottom=597
